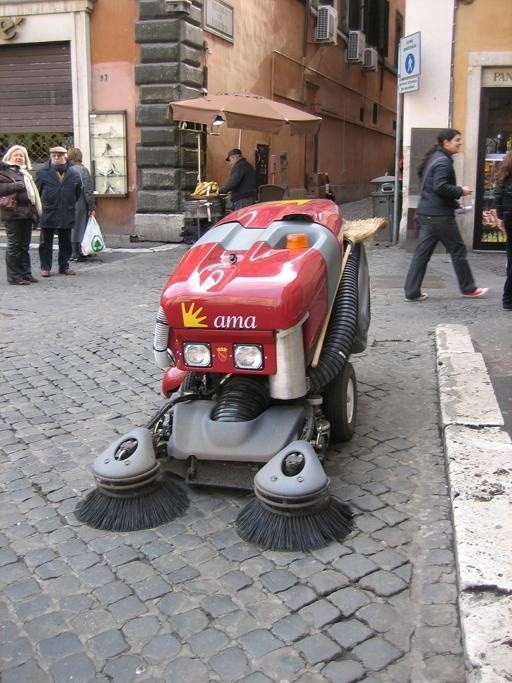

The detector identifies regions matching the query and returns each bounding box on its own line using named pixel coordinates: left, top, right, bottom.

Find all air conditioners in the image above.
left=362, top=48, right=378, bottom=70
left=348, top=30, right=366, bottom=64
left=317, top=5, right=338, bottom=45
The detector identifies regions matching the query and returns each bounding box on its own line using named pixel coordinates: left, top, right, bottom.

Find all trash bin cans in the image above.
left=370, top=183, right=402, bottom=242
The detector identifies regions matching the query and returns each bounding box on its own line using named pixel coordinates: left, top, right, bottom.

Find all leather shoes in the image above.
left=59, top=269, right=76, bottom=275
left=41, top=270, right=49, bottom=276
left=9, top=276, right=38, bottom=285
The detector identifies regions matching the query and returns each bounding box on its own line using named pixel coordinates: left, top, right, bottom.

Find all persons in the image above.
left=404, top=130, right=490, bottom=302
left=219, top=149, right=257, bottom=211
left=495, top=150, right=511, bottom=309
left=68, top=148, right=96, bottom=262
left=34, top=146, right=82, bottom=277
left=0, top=145, right=41, bottom=285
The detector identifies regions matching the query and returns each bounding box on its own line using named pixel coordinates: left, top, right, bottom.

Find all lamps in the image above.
left=212, top=115, right=225, bottom=126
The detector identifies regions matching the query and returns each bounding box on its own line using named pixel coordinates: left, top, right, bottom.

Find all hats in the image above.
left=224, top=149, right=241, bottom=161
left=49, top=147, right=68, bottom=153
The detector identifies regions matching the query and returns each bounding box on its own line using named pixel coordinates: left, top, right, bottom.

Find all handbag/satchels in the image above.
left=0, top=192, right=16, bottom=209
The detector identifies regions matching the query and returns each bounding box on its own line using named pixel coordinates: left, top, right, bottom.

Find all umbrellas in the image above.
left=167, top=88, right=322, bottom=149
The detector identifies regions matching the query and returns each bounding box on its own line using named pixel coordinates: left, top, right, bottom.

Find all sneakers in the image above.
left=460, top=288, right=488, bottom=298
left=404, top=293, right=428, bottom=302
